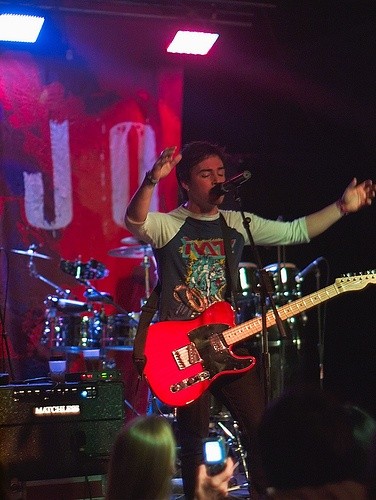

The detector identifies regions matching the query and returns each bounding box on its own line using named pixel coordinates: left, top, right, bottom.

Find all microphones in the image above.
left=295, top=256, right=324, bottom=283
left=214, top=171, right=252, bottom=196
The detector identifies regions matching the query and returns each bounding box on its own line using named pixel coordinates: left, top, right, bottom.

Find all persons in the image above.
left=124, top=140, right=376, bottom=499
left=249, top=385, right=376, bottom=500
left=105, top=414, right=177, bottom=500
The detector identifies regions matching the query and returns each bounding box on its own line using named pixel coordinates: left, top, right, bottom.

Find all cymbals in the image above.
left=108, top=236, right=154, bottom=259
left=8, top=248, right=52, bottom=260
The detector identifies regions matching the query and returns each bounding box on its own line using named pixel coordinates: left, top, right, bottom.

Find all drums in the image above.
left=231, top=263, right=262, bottom=301
left=263, top=263, right=303, bottom=304
left=235, top=298, right=291, bottom=348
left=108, top=311, right=139, bottom=352
left=55, top=316, right=107, bottom=355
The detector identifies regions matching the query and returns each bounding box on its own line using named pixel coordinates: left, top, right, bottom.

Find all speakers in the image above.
left=0, top=380, right=125, bottom=489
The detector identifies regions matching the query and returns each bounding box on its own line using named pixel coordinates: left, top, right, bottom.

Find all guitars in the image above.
left=133, top=269, right=376, bottom=411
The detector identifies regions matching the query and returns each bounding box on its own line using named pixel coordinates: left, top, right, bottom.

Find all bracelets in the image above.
left=337, top=197, right=349, bottom=216
left=145, top=170, right=159, bottom=186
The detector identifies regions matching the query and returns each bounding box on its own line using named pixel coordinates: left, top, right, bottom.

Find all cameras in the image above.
left=203, top=438, right=226, bottom=478
